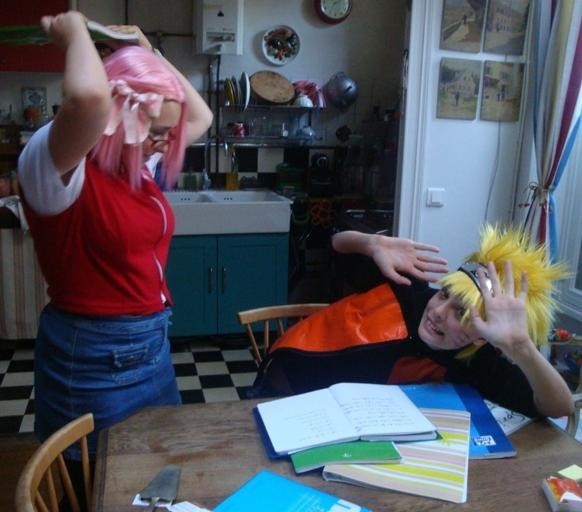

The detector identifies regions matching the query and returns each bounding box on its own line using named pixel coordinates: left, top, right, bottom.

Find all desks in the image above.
left=88, top=396, right=582, bottom=512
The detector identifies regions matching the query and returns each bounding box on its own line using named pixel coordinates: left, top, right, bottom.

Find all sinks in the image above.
left=154, top=188, right=218, bottom=236
left=205, top=187, right=294, bottom=235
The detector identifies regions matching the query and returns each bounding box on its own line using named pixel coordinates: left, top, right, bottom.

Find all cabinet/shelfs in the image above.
left=163, top=232, right=292, bottom=342
left=216, top=102, right=330, bottom=150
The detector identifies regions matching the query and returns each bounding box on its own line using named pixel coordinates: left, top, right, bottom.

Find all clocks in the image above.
left=315, top=0, right=353, bottom=25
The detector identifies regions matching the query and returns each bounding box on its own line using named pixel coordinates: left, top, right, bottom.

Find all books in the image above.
left=542, top=476, right=582, bottom=512
left=210, top=382, right=516, bottom=511
left=86, top=20, right=139, bottom=59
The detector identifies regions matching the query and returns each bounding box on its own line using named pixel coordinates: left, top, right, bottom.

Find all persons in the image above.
left=246, top=221, right=575, bottom=422
left=18, top=10, right=215, bottom=512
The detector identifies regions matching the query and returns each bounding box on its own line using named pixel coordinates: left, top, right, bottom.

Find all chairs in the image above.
left=236, top=303, right=332, bottom=368
left=12, top=412, right=97, bottom=512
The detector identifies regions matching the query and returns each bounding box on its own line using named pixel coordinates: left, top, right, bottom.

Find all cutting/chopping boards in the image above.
left=250, top=70, right=296, bottom=105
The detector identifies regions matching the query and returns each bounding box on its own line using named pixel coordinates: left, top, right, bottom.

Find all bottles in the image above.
left=227, top=145, right=239, bottom=190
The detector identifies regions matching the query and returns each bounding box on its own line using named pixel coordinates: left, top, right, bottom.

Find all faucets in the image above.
left=200, top=134, right=230, bottom=190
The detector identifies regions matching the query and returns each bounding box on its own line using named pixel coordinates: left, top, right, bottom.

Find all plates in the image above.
left=223, top=72, right=251, bottom=112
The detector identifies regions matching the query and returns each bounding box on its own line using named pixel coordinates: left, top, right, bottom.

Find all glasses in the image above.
left=147, top=134, right=176, bottom=148
